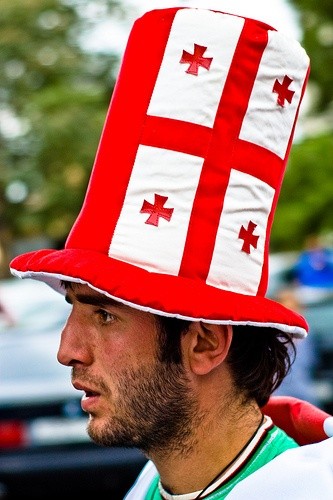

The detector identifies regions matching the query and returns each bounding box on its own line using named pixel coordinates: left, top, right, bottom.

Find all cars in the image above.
left=1, top=276, right=149, bottom=500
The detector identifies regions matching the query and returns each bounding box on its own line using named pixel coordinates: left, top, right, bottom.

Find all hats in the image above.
left=8, top=5, right=309, bottom=338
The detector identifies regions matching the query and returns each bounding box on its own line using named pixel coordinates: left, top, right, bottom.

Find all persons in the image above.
left=8, top=6, right=333, bottom=500
left=274, top=233, right=333, bottom=317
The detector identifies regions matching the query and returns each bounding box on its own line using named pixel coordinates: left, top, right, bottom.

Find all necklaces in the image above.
left=194, top=413, right=265, bottom=500
